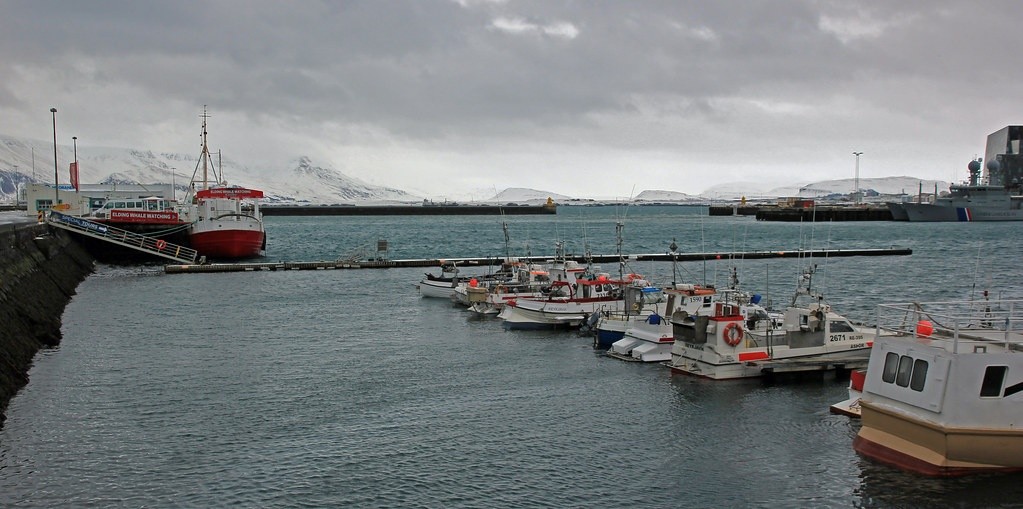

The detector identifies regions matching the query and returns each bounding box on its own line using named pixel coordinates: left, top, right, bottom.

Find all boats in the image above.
left=829, top=243, right=1023, bottom=479
left=884, top=154, right=1023, bottom=221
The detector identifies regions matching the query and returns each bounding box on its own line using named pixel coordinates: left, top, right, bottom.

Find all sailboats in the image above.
left=179, top=104, right=266, bottom=259
left=420, top=197, right=897, bottom=379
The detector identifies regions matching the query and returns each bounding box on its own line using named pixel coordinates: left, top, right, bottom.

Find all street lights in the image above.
left=852, top=152, right=863, bottom=205
left=50, top=108, right=59, bottom=205
left=171, top=167, right=176, bottom=200
left=13, top=166, right=19, bottom=205
left=73, top=136, right=78, bottom=193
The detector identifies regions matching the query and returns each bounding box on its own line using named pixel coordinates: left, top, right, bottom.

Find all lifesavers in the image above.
left=611, top=292, right=618, bottom=299
left=495, top=285, right=508, bottom=294
left=724, top=322, right=743, bottom=347
left=156, top=240, right=166, bottom=249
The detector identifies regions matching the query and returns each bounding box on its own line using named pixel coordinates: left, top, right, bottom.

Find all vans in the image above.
left=92, top=199, right=144, bottom=219
